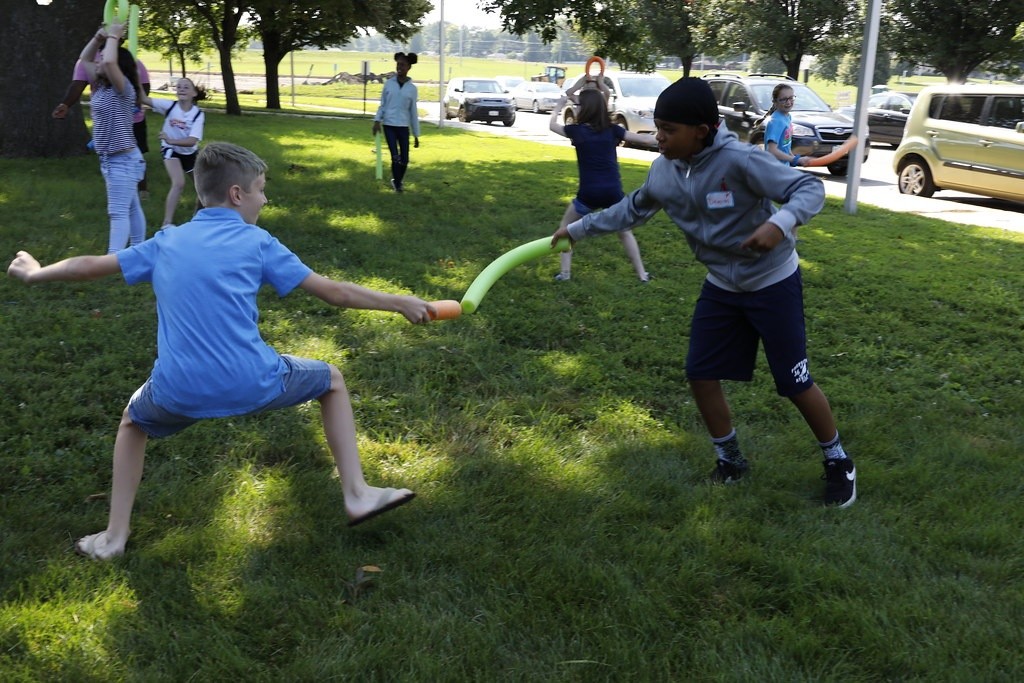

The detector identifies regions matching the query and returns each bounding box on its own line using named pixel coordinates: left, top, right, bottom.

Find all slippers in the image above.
left=74, top=530, right=105, bottom=565
left=346, top=488, right=418, bottom=528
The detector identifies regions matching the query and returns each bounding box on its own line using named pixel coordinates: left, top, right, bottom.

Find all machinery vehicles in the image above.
left=531, top=66, right=568, bottom=88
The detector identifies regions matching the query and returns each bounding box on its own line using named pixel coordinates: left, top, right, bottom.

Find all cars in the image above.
left=513, top=82, right=568, bottom=113
left=562, top=70, right=674, bottom=152
left=833, top=91, right=915, bottom=147
left=891, top=85, right=1024, bottom=212
left=496, top=76, right=527, bottom=91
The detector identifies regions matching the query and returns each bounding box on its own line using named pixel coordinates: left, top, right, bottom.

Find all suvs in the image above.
left=702, top=71, right=875, bottom=175
left=443, top=77, right=517, bottom=127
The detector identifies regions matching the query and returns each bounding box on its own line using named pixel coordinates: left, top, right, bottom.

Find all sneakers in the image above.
left=823, top=450, right=856, bottom=509
left=555, top=272, right=570, bottom=281
left=638, top=272, right=648, bottom=282
left=709, top=458, right=752, bottom=486
left=391, top=179, right=404, bottom=193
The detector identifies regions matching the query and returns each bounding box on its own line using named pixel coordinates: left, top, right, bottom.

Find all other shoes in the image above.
left=139, top=191, right=150, bottom=201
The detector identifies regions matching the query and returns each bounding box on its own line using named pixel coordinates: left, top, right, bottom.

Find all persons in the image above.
left=550, top=76, right=859, bottom=513
left=53, top=22, right=207, bottom=254
left=752, top=83, right=811, bottom=240
left=9, top=142, right=439, bottom=563
left=372, top=52, right=421, bottom=194
left=550, top=74, right=659, bottom=282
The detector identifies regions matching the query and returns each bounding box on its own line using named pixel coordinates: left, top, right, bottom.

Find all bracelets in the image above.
left=793, top=154, right=801, bottom=167
left=106, top=35, right=117, bottom=39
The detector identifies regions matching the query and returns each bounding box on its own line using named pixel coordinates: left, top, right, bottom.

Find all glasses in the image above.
left=776, top=95, right=796, bottom=103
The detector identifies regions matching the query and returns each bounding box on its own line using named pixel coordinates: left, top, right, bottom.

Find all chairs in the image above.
left=728, top=85, right=749, bottom=110
left=943, top=98, right=1020, bottom=128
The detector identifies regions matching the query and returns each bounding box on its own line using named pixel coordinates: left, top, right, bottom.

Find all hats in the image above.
left=653, top=77, right=719, bottom=125
